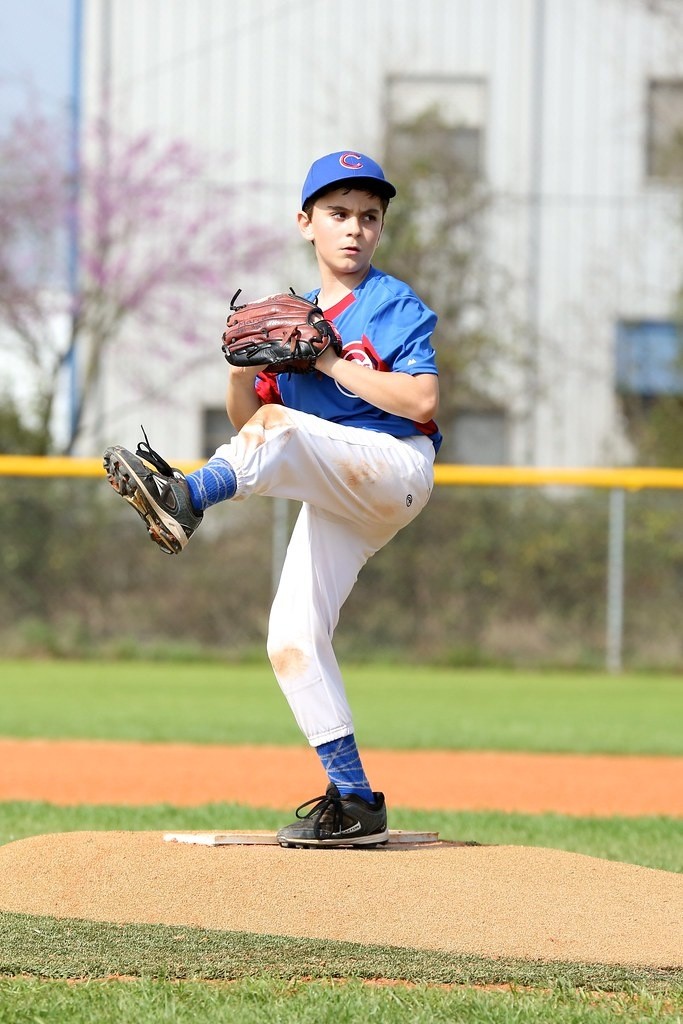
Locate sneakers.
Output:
[103,425,204,555]
[276,782,389,849]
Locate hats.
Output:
[302,150,396,211]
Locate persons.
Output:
[102,151,443,848]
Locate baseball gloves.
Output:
[220,286,343,373]
